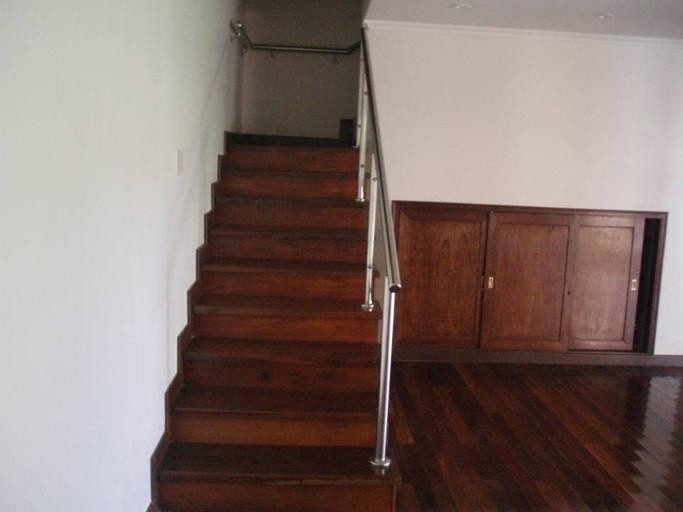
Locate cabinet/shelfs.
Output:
[391,201,487,349]
[479,203,668,355]
